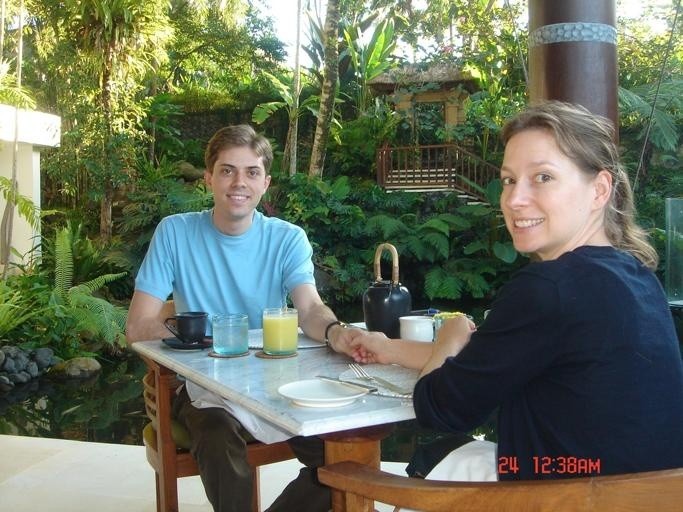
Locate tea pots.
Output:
[361,242,410,340]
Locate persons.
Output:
[124,124,374,512]
[348,99,683,485]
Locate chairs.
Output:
[317,460,682,512]
[127,300,298,512]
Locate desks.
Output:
[131,321,418,512]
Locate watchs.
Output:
[325,320,347,347]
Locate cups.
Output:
[163,312,208,345]
[432,312,472,338]
[211,313,250,356]
[398,314,437,343]
[261,308,300,356]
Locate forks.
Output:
[346,361,414,395]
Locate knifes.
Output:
[313,375,380,395]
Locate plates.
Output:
[160,336,213,352]
[276,378,367,409]
[337,363,420,399]
[246,326,332,350]
